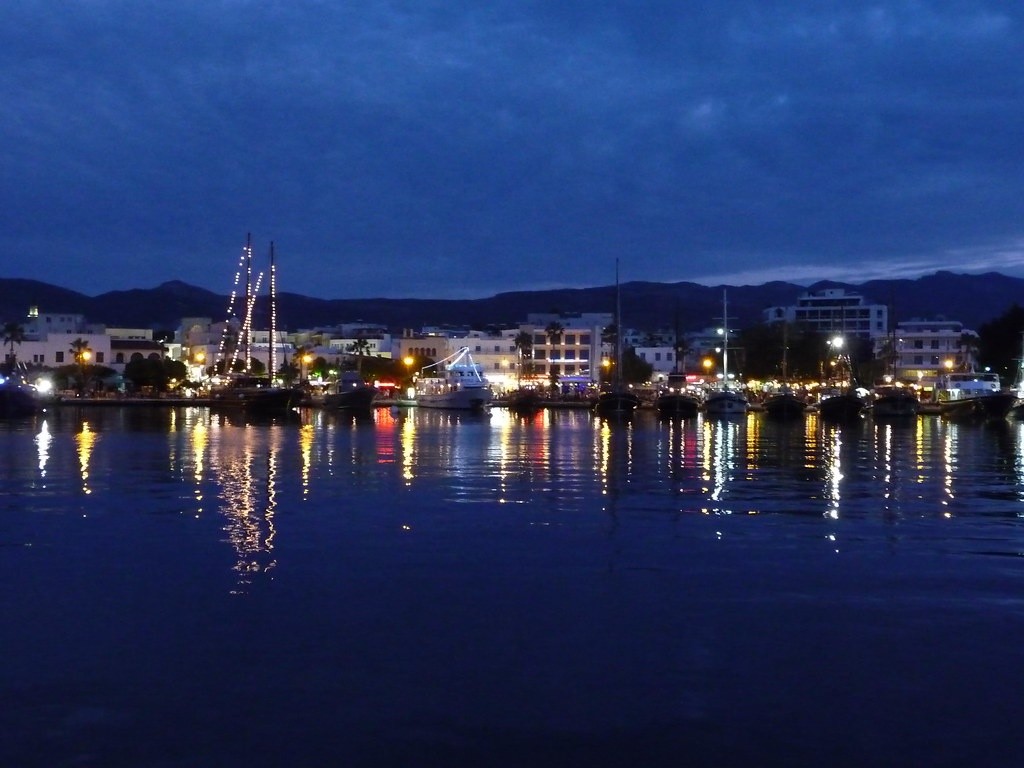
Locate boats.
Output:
[763,393,808,419]
[820,393,866,424]
[592,390,642,413]
[939,393,1019,423]
[653,389,699,419]
[701,385,748,415]
[873,395,919,418]
[323,386,379,414]
[232,388,305,417]
[416,346,495,408]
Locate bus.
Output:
[937,373,1002,399]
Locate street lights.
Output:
[405,356,414,376]
[704,357,716,382]
[602,359,617,381]
[824,336,845,374]
[9,305,39,355]
[77,350,92,390]
[299,354,314,385]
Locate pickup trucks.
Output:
[54,389,79,399]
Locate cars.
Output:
[0,370,38,422]
[140,385,160,396]
